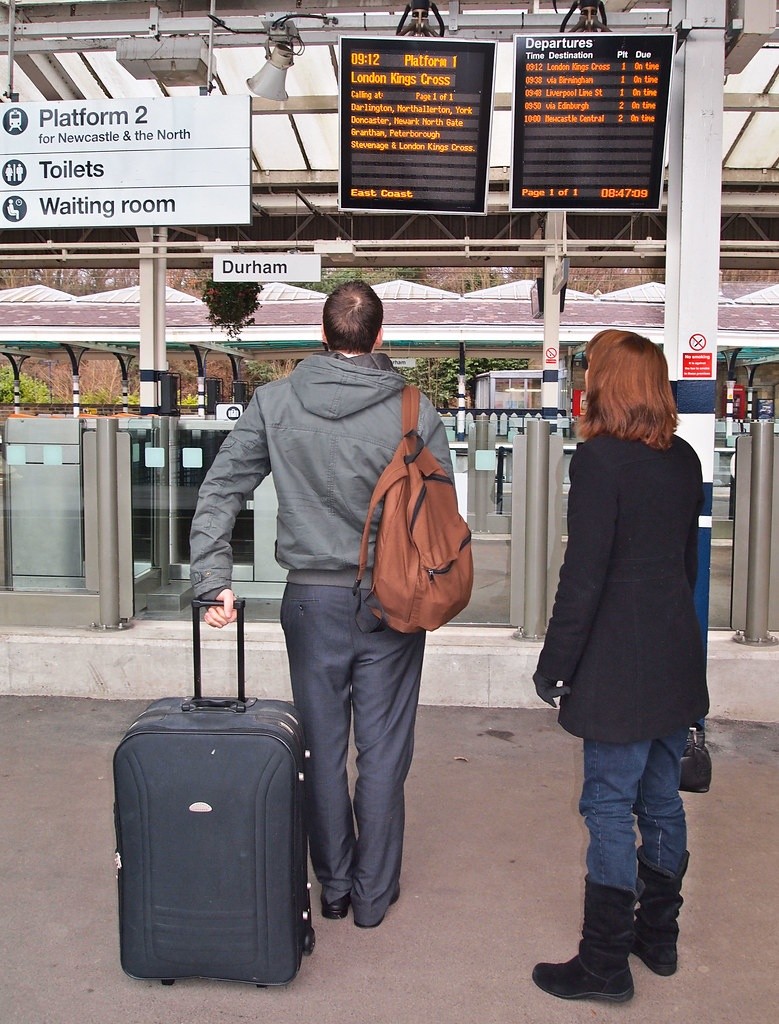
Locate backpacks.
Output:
[354,383,473,636]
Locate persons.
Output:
[190,281,455,927]
[530,331,712,998]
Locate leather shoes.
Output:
[322,893,351,920]
[355,882,401,926]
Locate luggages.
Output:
[112,599,316,985]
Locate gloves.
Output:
[532,673,570,707]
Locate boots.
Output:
[531,873,646,1001]
[631,845,691,976]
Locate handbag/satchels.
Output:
[677,741,715,791]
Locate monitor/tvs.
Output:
[509,32,677,212]
[335,34,499,216]
